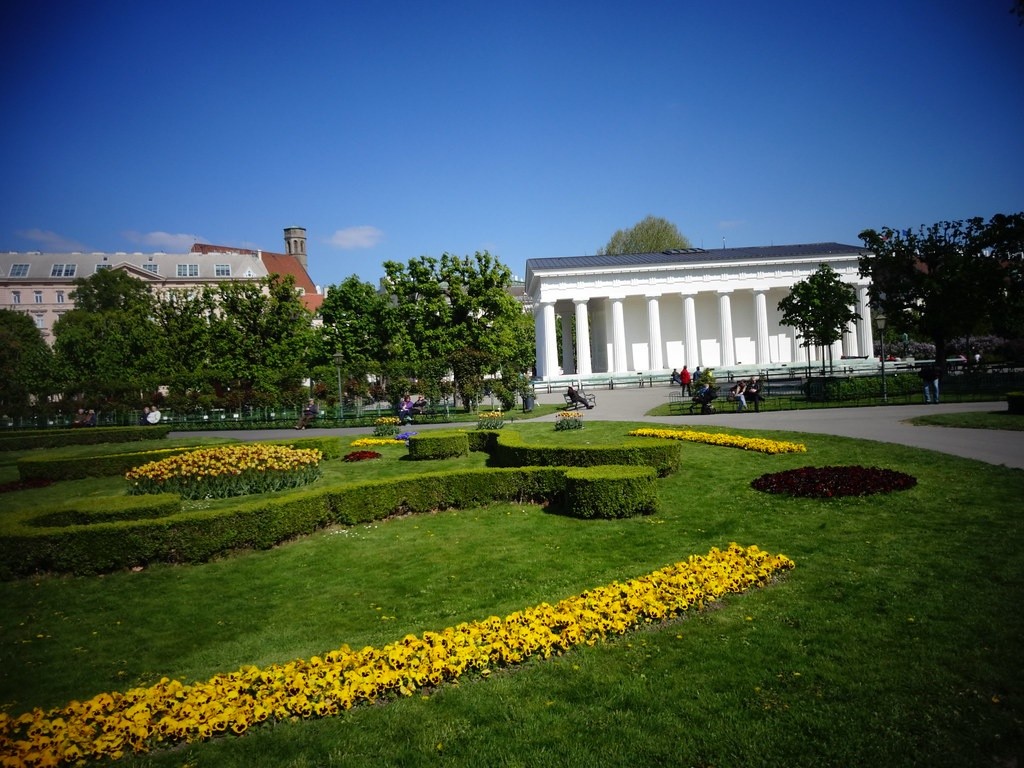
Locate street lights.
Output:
[333,350,345,419]
[874,314,888,403]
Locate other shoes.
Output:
[406,416,412,421]
[689,395,692,397]
[587,405,594,409]
[405,423,411,426]
[755,409,759,412]
[744,406,748,409]
[737,409,741,413]
[400,417,404,420]
[759,397,765,401]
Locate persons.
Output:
[342,392,358,418]
[294,399,320,430]
[693,367,702,381]
[74,409,87,428]
[923,363,945,404]
[567,386,594,409]
[672,369,681,384]
[680,366,692,396]
[731,377,765,414]
[405,396,427,427]
[84,410,97,428]
[694,384,709,398]
[399,395,413,426]
[140,404,162,426]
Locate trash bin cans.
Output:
[524,393,534,409]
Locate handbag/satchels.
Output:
[727,394,735,401]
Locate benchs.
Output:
[563,389,595,411]
[520,358,1024,394]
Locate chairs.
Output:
[0,377,1024,432]
